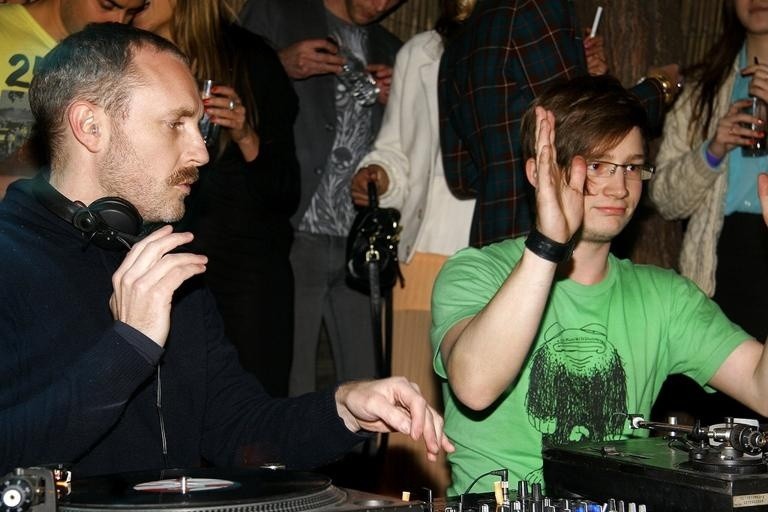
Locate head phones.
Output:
[30,168,144,254]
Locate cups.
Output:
[738,97,767,158]
[195,79,228,147]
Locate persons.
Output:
[1,21,456,496]
[426,74,768,498]
[343,2,476,496]
[234,0,411,402]
[644,0,768,428]
[131,0,306,400]
[433,2,688,251]
[2,0,151,204]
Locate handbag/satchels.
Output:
[344,182,402,296]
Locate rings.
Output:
[727,125,735,134]
[226,100,237,111]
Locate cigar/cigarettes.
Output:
[589,5,604,40]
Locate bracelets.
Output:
[523,226,577,266]
[649,74,674,109]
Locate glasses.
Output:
[586,161,655,180]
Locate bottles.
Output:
[327,38,380,106]
